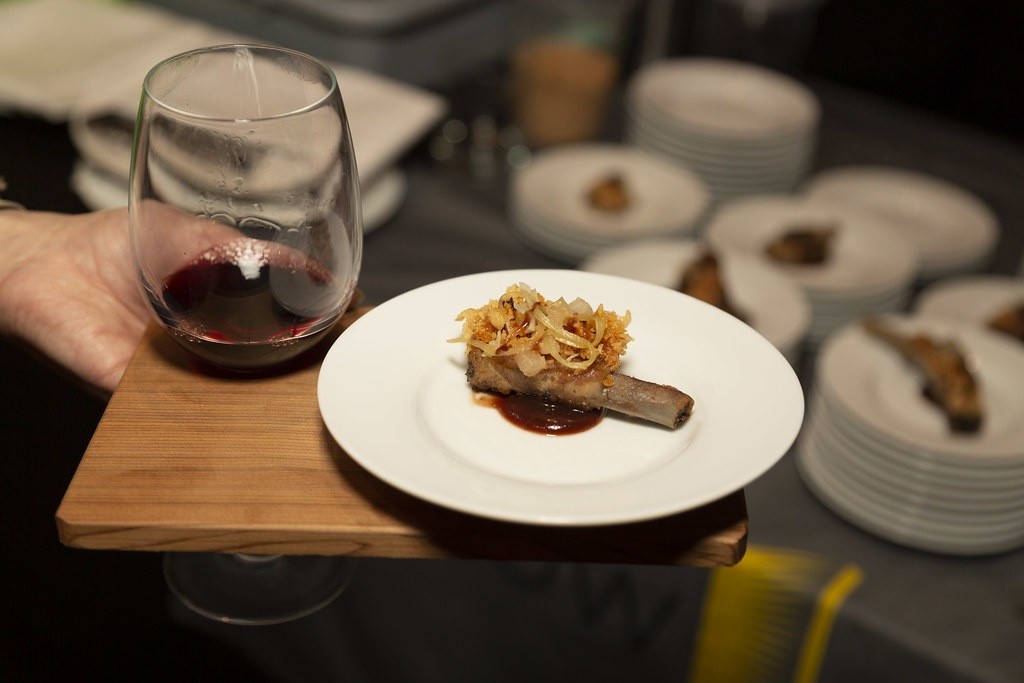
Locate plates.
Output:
[70,59,1024,555]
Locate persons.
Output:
[1,177,363,394]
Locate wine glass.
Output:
[128,46,362,624]
